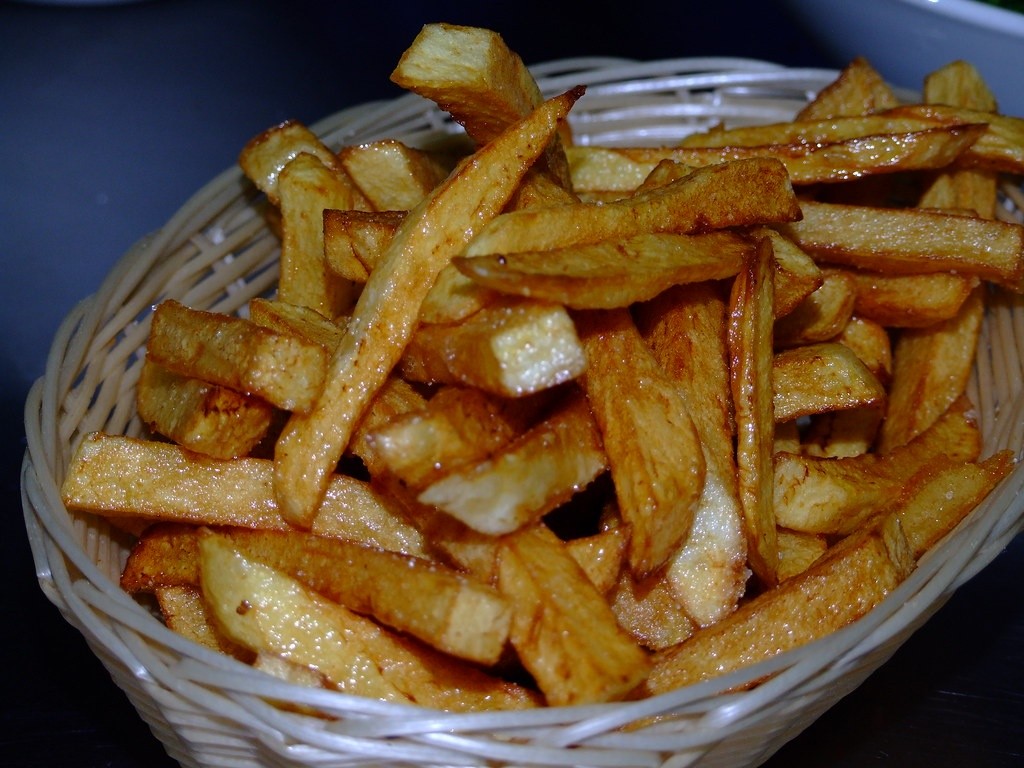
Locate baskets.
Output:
[16,55,1024,768]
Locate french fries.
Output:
[62,23,1024,715]
